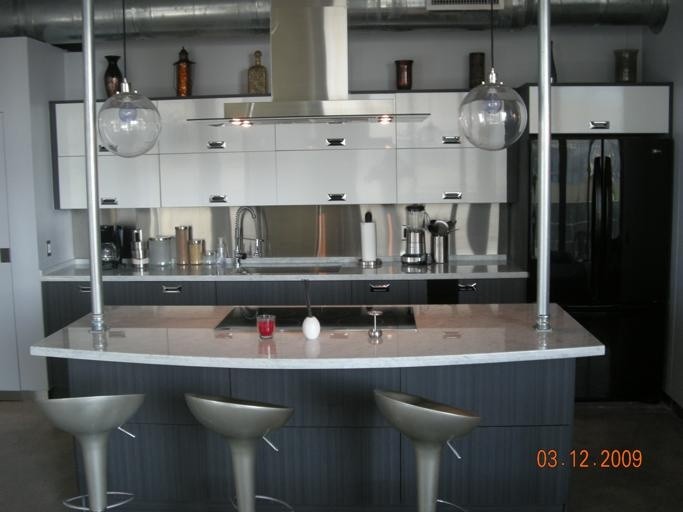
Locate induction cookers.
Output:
[214,306,417,332]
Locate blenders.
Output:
[403,205,430,263]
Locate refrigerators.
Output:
[527,136,674,405]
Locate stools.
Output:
[183,392,297,510]
[43,391,147,509]
[372,390,480,510]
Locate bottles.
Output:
[103,56,124,101]
[174,44,194,98]
[550,41,556,85]
[247,50,268,95]
[395,60,414,92]
[148,226,226,267]
[615,49,639,84]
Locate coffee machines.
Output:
[100,225,121,270]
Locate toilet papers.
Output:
[359,220,377,263]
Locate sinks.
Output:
[244,262,342,275]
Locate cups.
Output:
[256,315,277,340]
[469,52,485,89]
[432,234,449,264]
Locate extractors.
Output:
[184,0,432,124]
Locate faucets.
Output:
[233,206,256,266]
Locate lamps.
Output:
[456,2,530,151]
[97,2,159,157]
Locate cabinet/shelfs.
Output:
[510,80,670,136]
[44,283,215,397]
[353,280,524,306]
[49,88,509,208]
